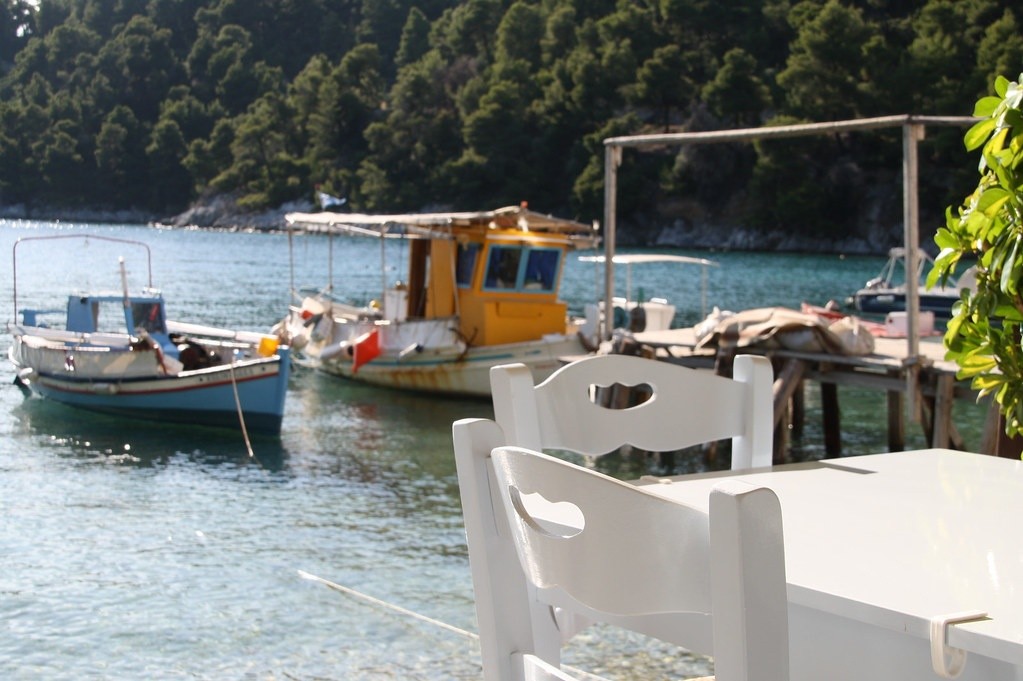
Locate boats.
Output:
[575,254,726,368]
[847,246,1001,330]
[267,199,602,395]
[7,234,292,437]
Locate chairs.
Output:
[490,353,776,470]
[452,416,787,681]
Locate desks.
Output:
[533,445,1023,681]
